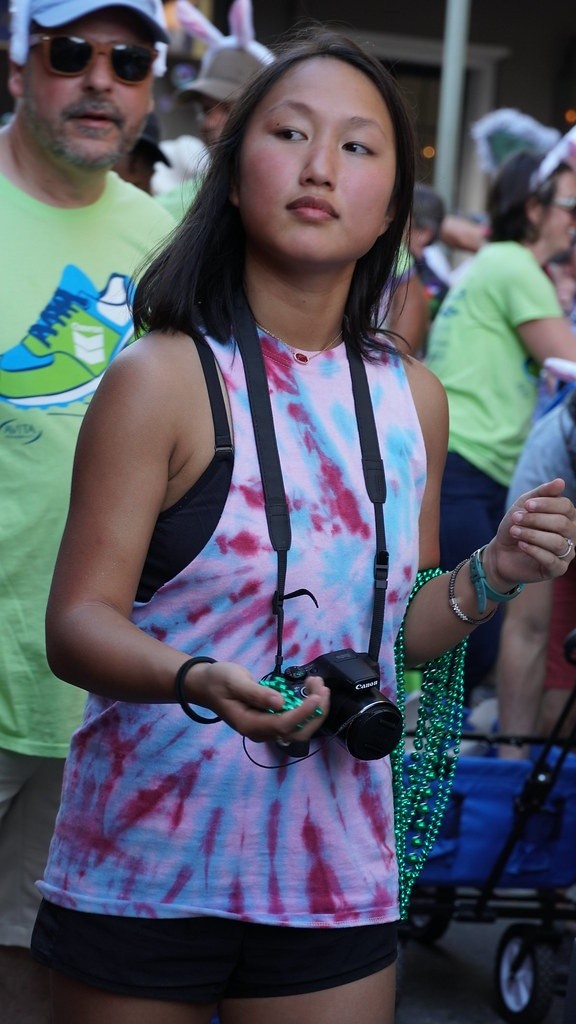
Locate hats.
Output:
[175,0,277,100]
[147,135,211,193]
[134,112,173,171]
[27,0,173,44]
[468,107,561,187]
[410,183,445,248]
[470,128,576,218]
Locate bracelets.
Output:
[448,559,497,624]
[470,544,524,615]
[176,656,223,724]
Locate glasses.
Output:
[29,33,160,85]
[550,197,576,215]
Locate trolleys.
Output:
[390,629,576,1024]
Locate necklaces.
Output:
[393,569,472,921]
[256,322,343,364]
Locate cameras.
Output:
[282,649,402,762]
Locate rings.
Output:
[557,538,574,559]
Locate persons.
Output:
[28,34,576,1024]
[368,108,576,762]
[0,0,280,1024]
